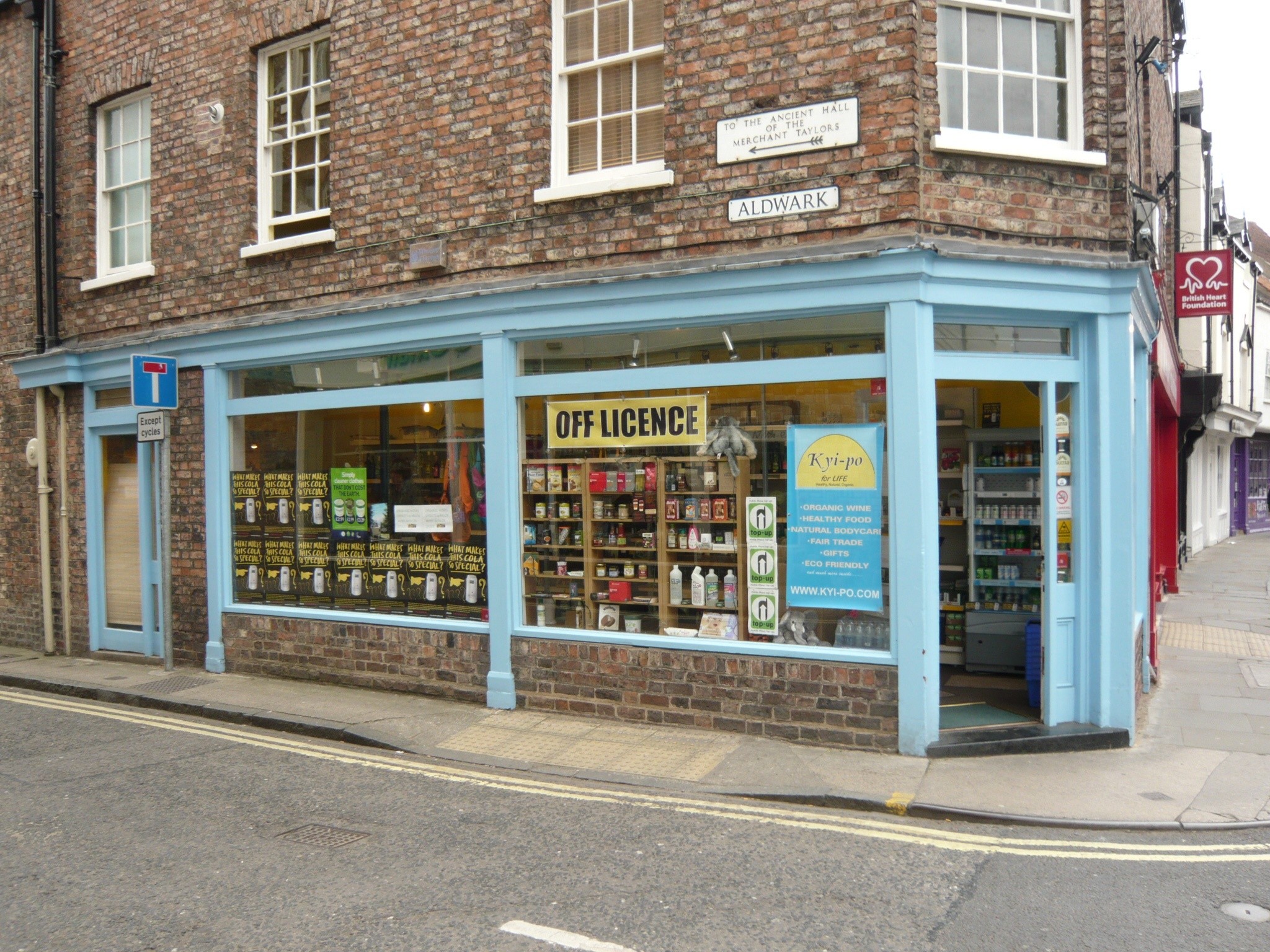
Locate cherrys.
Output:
[942,453,959,470]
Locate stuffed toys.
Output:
[696,415,758,477]
[772,608,832,646]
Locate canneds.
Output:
[426,573,437,600]
[728,497,736,519]
[279,499,289,524]
[387,571,397,598]
[535,502,582,519]
[313,499,324,524]
[351,569,362,596]
[248,565,258,590]
[976,476,1041,520]
[677,462,718,492]
[618,504,629,520]
[595,561,648,579]
[465,575,477,603]
[592,533,605,547]
[603,504,615,519]
[280,566,290,592]
[246,498,255,522]
[314,568,324,593]
[542,527,583,597]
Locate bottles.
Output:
[667,523,678,549]
[537,599,546,626]
[623,614,642,633]
[1032,527,1041,550]
[691,565,705,606]
[705,568,719,606]
[833,616,890,650]
[990,441,1041,467]
[678,529,688,549]
[724,569,738,608]
[975,528,1026,549]
[608,524,617,547]
[424,451,438,478]
[670,565,683,605]
[616,523,627,547]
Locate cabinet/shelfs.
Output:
[965,426,1043,676]
[522,416,965,666]
[351,434,460,487]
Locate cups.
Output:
[557,562,567,576]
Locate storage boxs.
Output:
[606,470,617,491]
[1024,616,1042,705]
[700,498,712,520]
[625,472,635,492]
[568,464,581,491]
[526,464,545,491]
[713,498,729,520]
[547,465,564,492]
[597,603,620,631]
[609,581,632,601]
[683,497,699,520]
[617,472,625,491]
[665,499,680,520]
[590,470,606,492]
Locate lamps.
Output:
[619,356,627,369]
[824,342,835,356]
[531,359,540,376]
[585,358,593,372]
[871,338,883,352]
[719,327,741,362]
[628,335,644,367]
[701,348,710,364]
[768,344,778,360]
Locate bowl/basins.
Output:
[664,627,698,637]
